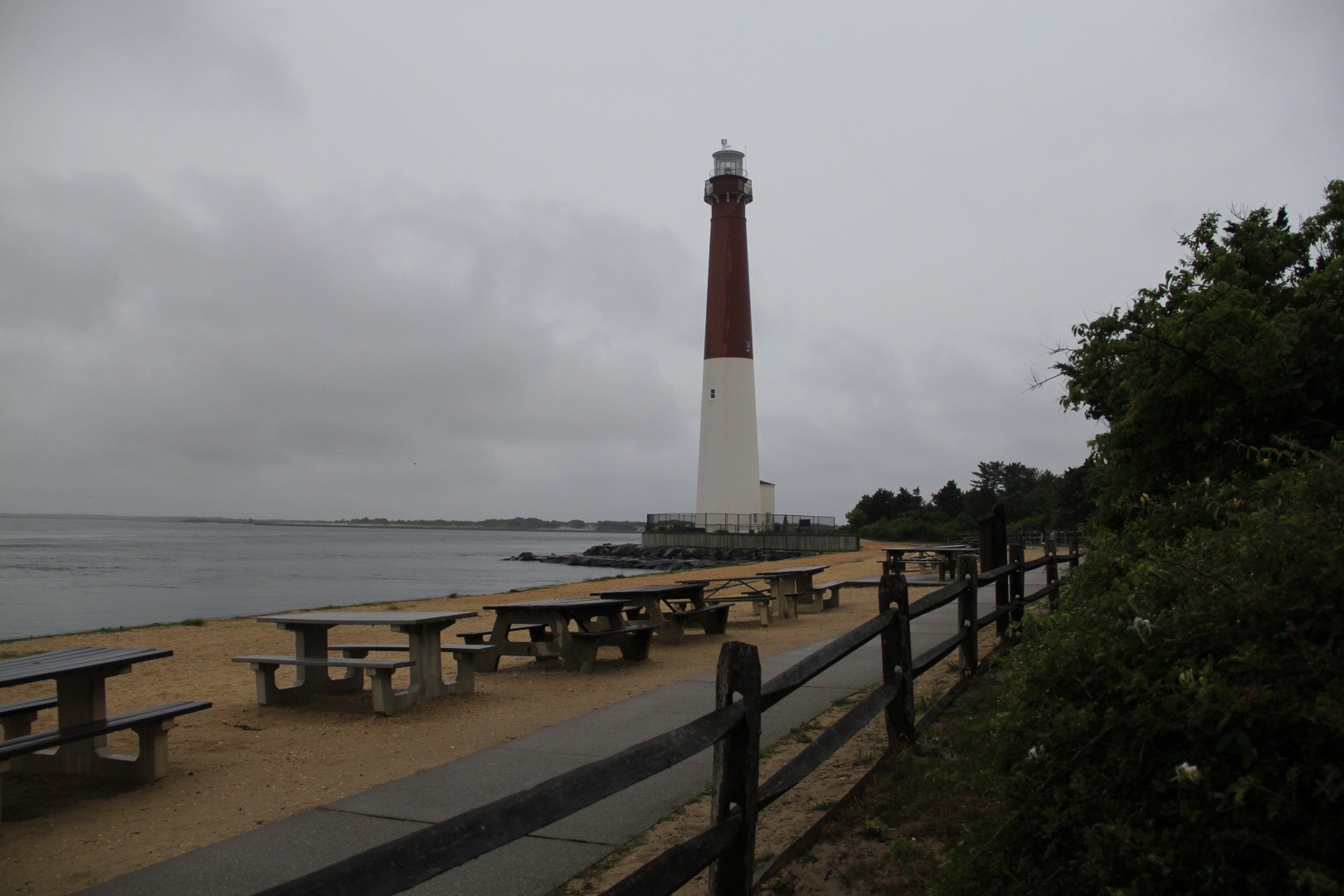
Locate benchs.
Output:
[455,624,548,672]
[0,699,213,782]
[230,655,416,715]
[808,580,846,612]
[622,607,642,620]
[741,587,772,612]
[0,696,58,739]
[784,592,804,617]
[668,596,776,627]
[876,559,956,581]
[912,554,940,560]
[568,624,659,671]
[327,642,494,700]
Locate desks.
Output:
[674,574,787,612]
[756,566,830,608]
[590,583,710,645]
[0,645,173,782]
[250,610,477,715]
[482,598,633,673]
[881,546,979,581]
